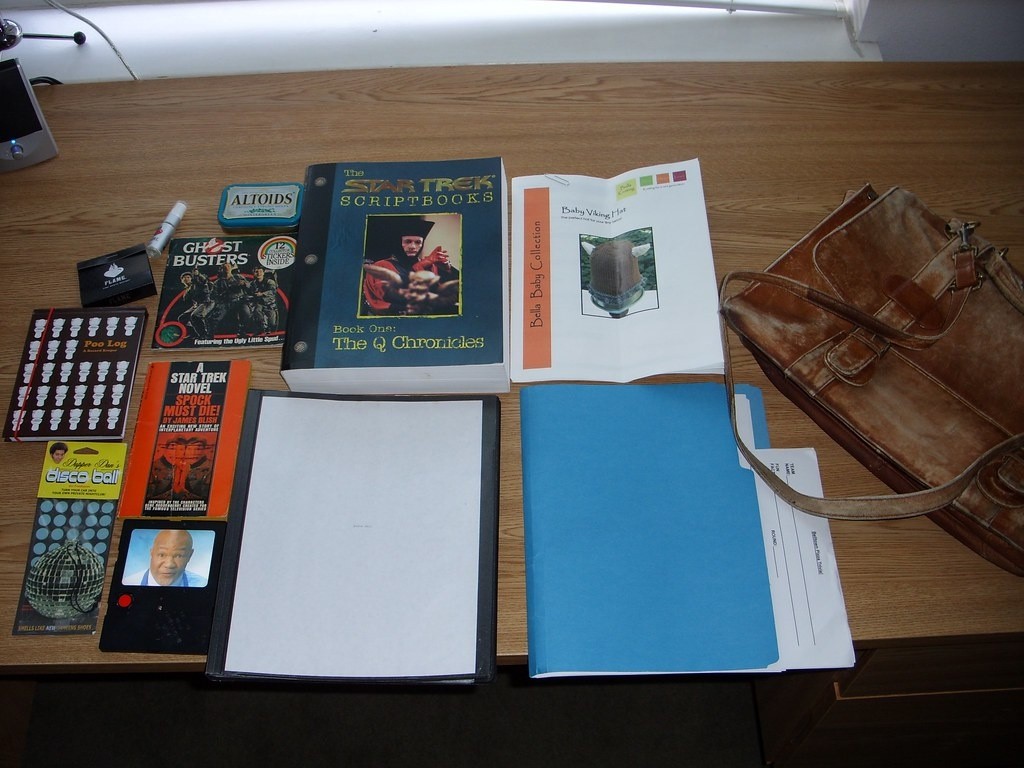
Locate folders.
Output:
[522,379,793,686]
[203,388,501,690]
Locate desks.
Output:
[0,61,1024,768]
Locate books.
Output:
[148,233,298,351]
[117,359,252,524]
[2,307,149,442]
[11,499,118,635]
[205,389,501,691]
[278,157,512,396]
[509,158,724,386]
[37,439,128,500]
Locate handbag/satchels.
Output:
[723,184,1023,593]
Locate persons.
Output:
[179,263,278,342]
[364,220,459,315]
[121,530,209,586]
